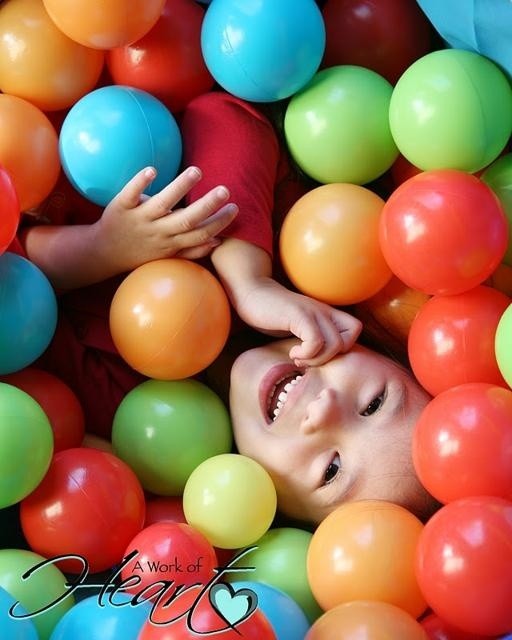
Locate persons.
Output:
[1,0,512,640]
